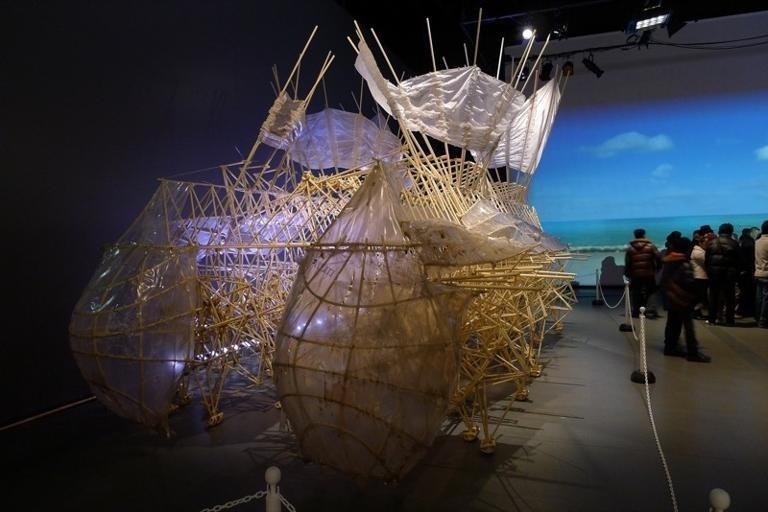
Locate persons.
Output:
[623,228,661,321]
[656,238,713,362]
[664,220,768,329]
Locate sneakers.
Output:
[694,312,768,328]
[663,344,712,362]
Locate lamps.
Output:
[510,49,606,81]
